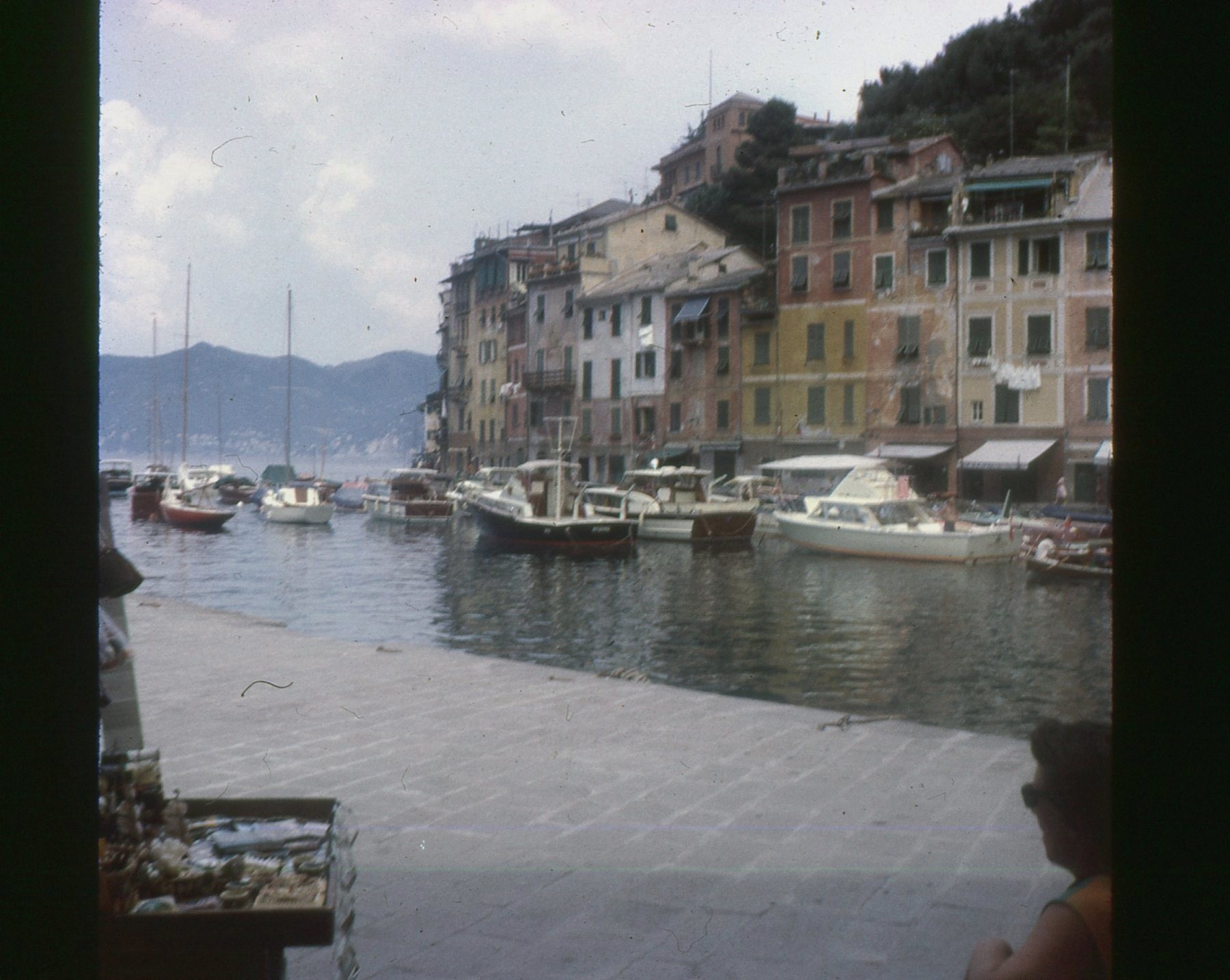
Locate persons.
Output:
[964,720,1111,979]
[1054,473,1067,505]
[941,498,956,533]
[1023,516,1112,571]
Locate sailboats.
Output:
[126,263,344,525]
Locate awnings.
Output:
[867,442,954,461]
[759,454,889,472]
[1094,439,1113,468]
[957,438,1064,469]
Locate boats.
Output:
[258,487,336,525]
[362,467,454,519]
[579,458,762,542]
[98,458,134,496]
[460,415,640,547]
[1021,459,1114,582]
[445,465,517,515]
[709,472,805,529]
[328,477,387,509]
[767,463,1024,563]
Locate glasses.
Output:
[1021,783,1050,808]
